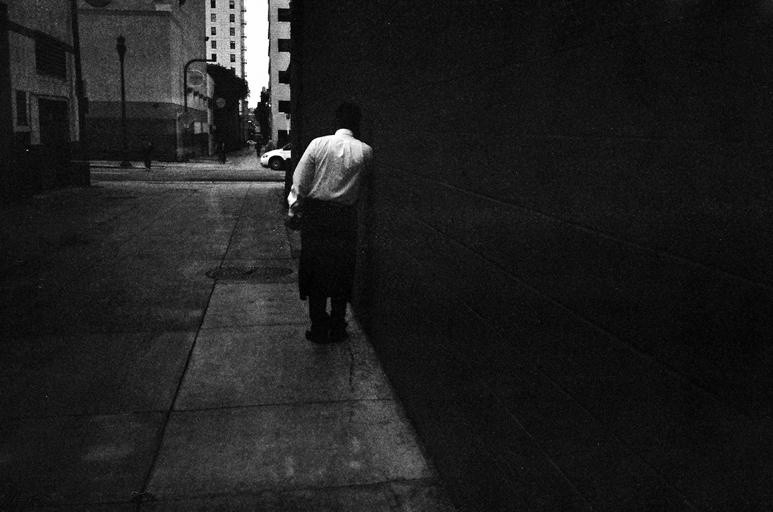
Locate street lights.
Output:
[115,33,135,168]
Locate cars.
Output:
[259,143,292,170]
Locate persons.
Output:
[286,105,375,345]
